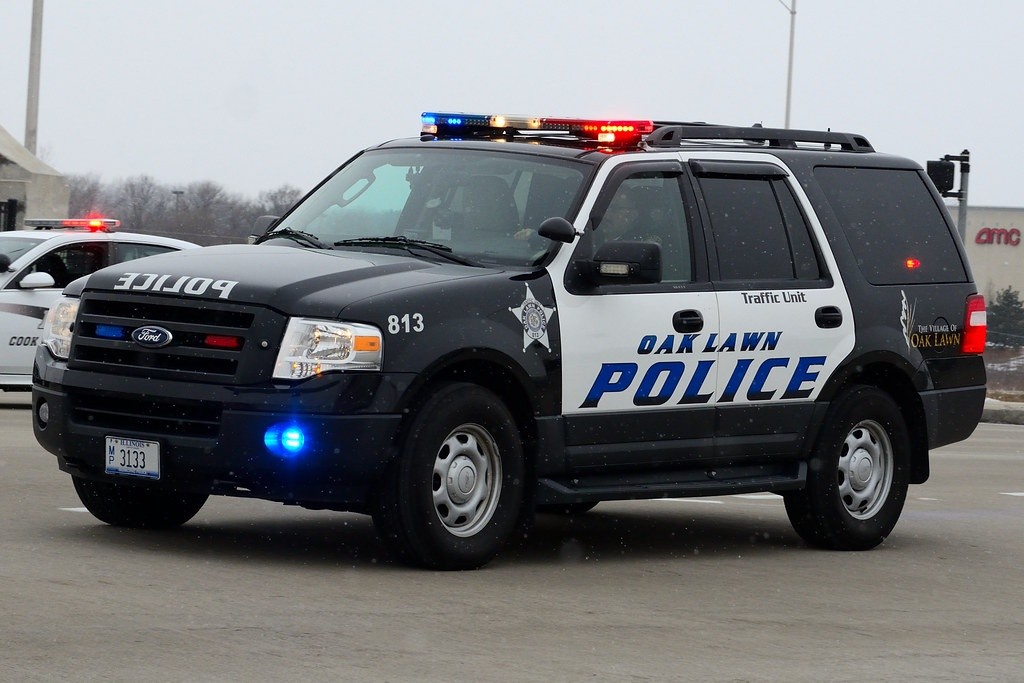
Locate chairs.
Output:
[633,185,685,280]
[452,175,521,249]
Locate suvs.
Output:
[28,111,988,573]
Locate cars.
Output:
[0,217,206,390]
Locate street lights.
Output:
[171,190,184,223]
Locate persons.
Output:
[513,187,669,269]
